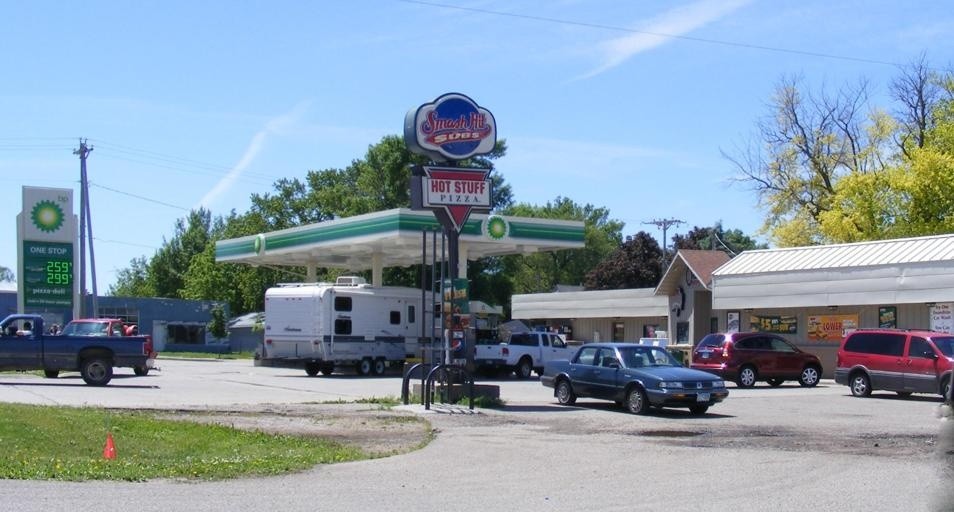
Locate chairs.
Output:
[603,357,615,368]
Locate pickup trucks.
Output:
[0,315,156,387]
[473,332,579,380]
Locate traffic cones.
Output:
[103,432,119,461]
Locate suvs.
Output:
[692,332,823,390]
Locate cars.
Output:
[540,343,729,417]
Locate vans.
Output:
[834,328,953,404]
